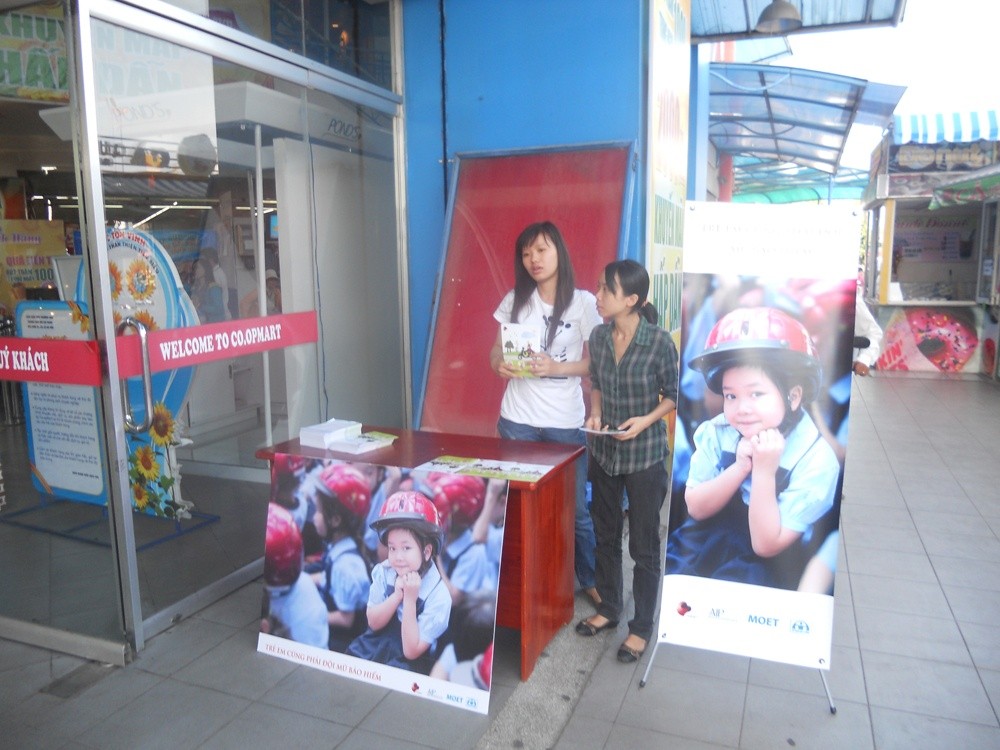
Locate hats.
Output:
[263,269,277,280]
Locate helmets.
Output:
[472,641,493,690]
[310,464,371,517]
[369,471,484,554]
[272,454,303,484]
[686,307,814,392]
[264,501,302,597]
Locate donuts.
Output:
[882,306,980,376]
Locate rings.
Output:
[862,371,865,376]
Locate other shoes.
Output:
[575,615,619,636]
[583,588,602,610]
[617,627,653,663]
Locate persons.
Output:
[179,249,231,328]
[576,259,678,664]
[855,295,886,377]
[489,220,604,614]
[664,260,852,597]
[261,451,508,692]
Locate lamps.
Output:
[754,0,802,37]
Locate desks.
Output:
[254,425,588,681]
